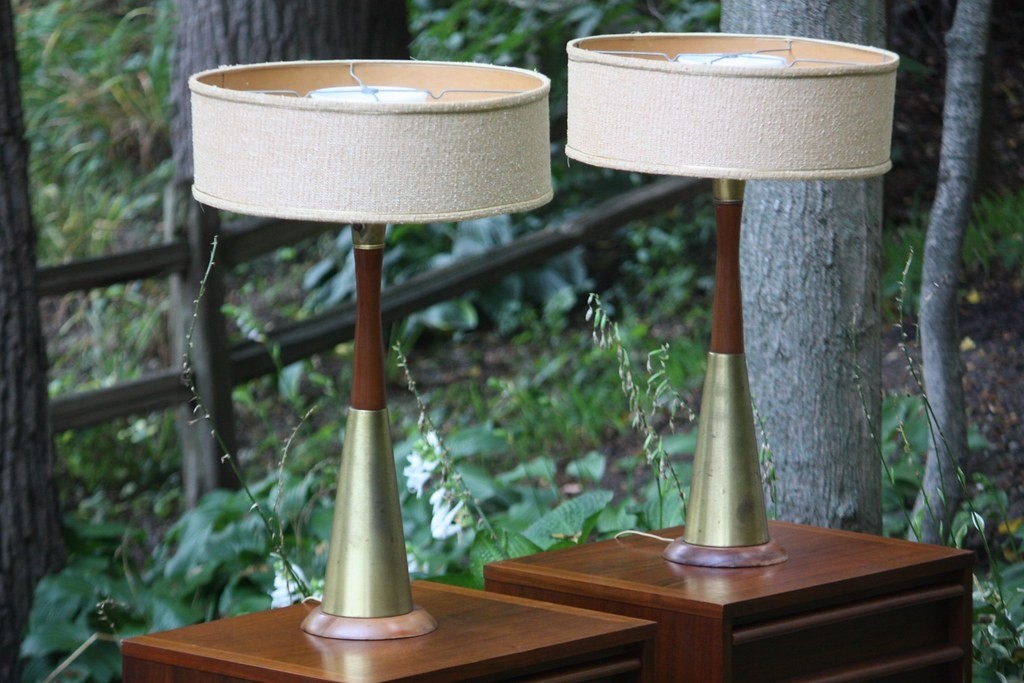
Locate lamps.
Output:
[565,33,901,570]
[186,57,553,641]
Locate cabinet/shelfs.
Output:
[482,519,974,683]
[121,578,658,683]
